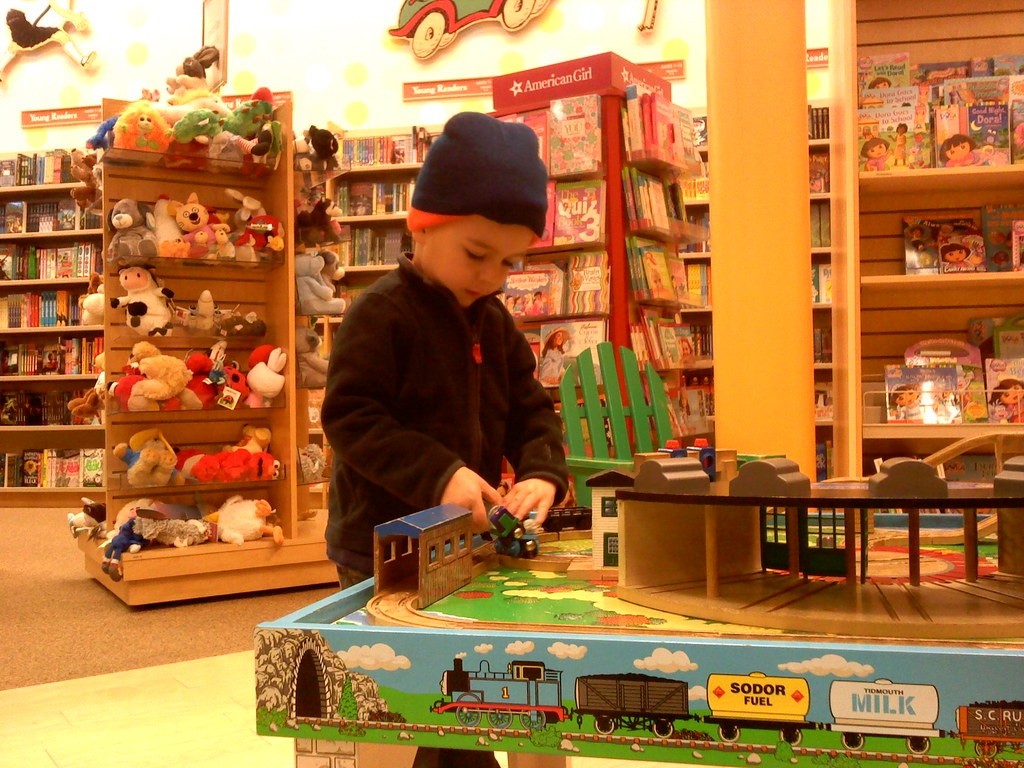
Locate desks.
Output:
[252,512,1024,768]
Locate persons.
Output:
[321,112,570,591]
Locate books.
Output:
[0,146,106,491]
[900,198,1024,277]
[885,358,1024,424]
[857,50,1024,170]
[496,85,832,443]
[333,125,443,317]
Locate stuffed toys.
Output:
[65,263,288,580]
[84,46,345,173]
[105,188,343,264]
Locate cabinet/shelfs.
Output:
[0,0,1024,607]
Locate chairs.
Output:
[558,341,674,508]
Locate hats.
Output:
[407,112,548,249]
